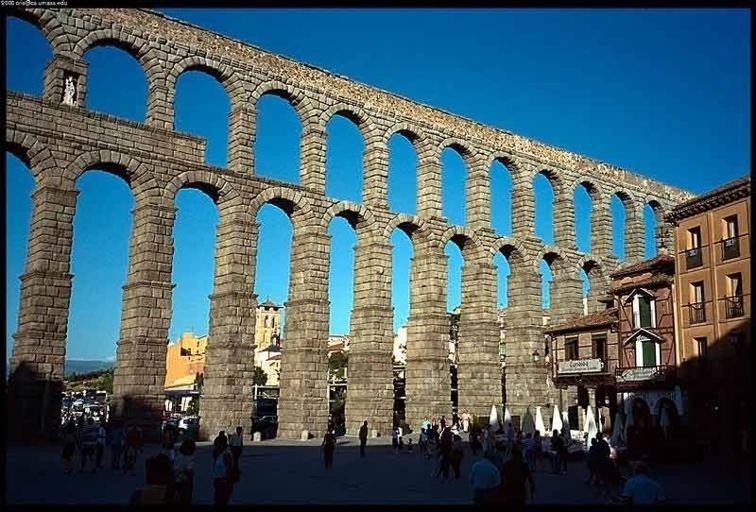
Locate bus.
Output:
[71,398,106,428]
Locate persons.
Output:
[185,347,193,355]
[357,419,369,458]
[388,409,756,512]
[47,404,243,508]
[319,424,336,471]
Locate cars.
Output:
[162,401,278,438]
